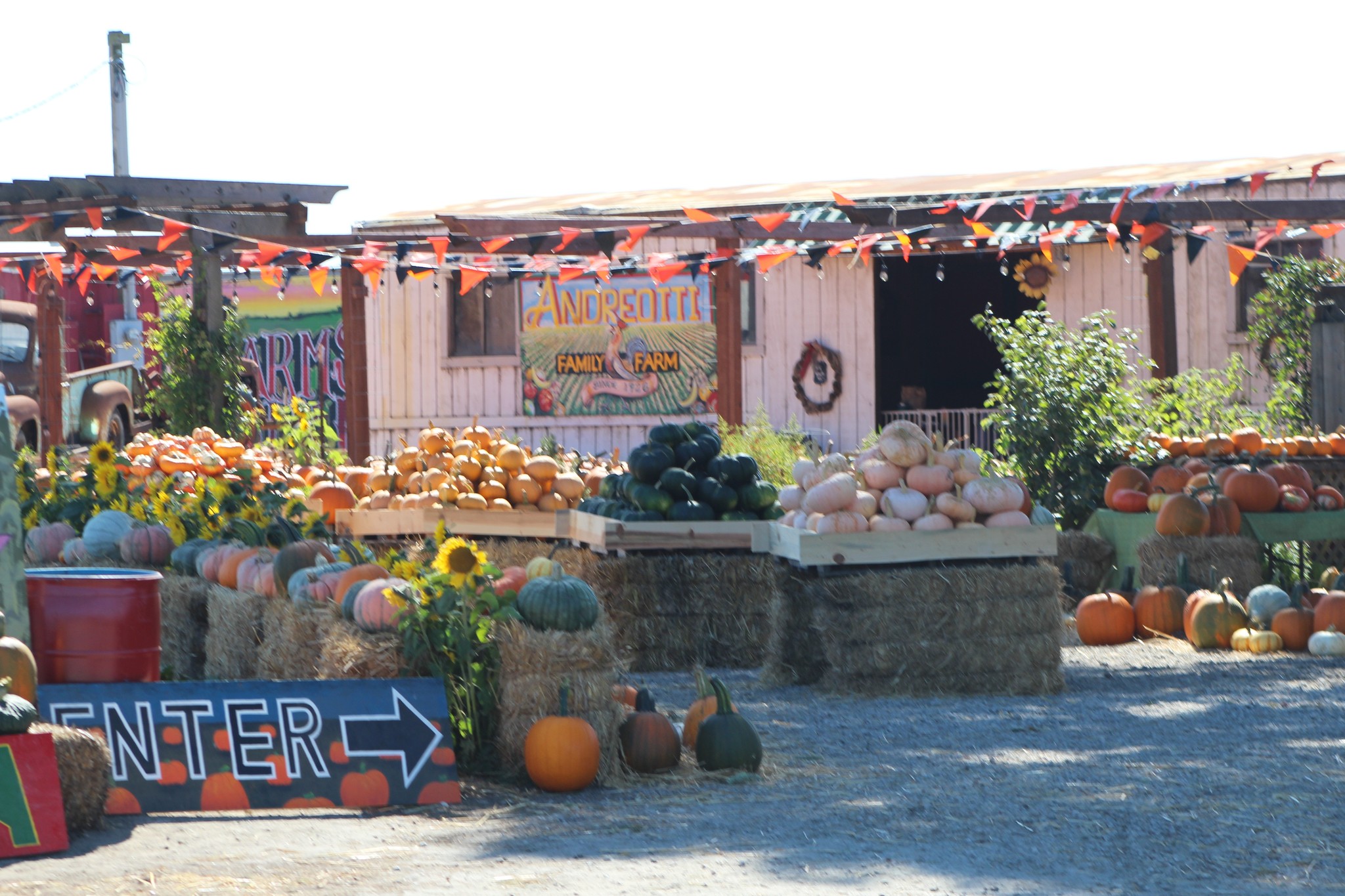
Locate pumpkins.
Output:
[1,418,1344,840]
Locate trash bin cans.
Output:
[23,566,164,684]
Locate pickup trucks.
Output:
[0,298,157,461]
